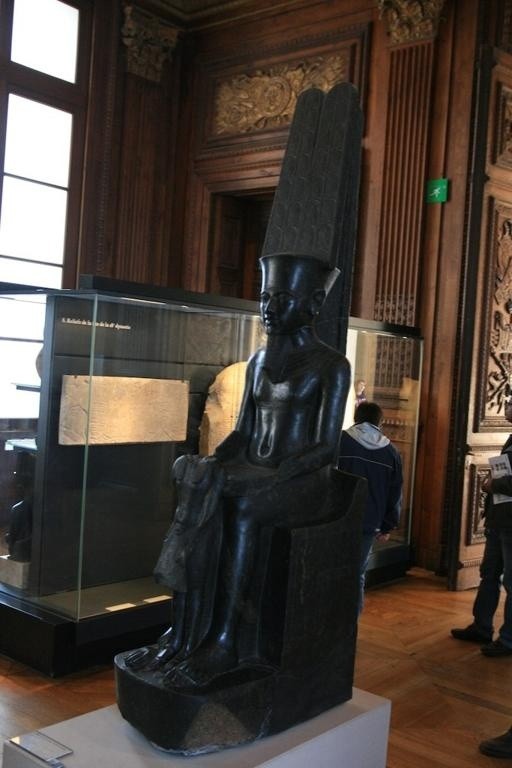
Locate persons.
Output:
[451,395,512,657]
[337,398,404,619]
[153,255,351,673]
[478,723,512,760]
[354,379,367,407]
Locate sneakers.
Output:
[479,640,512,657]
[479,728,511,759]
[450,623,494,644]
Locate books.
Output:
[488,454,512,505]
